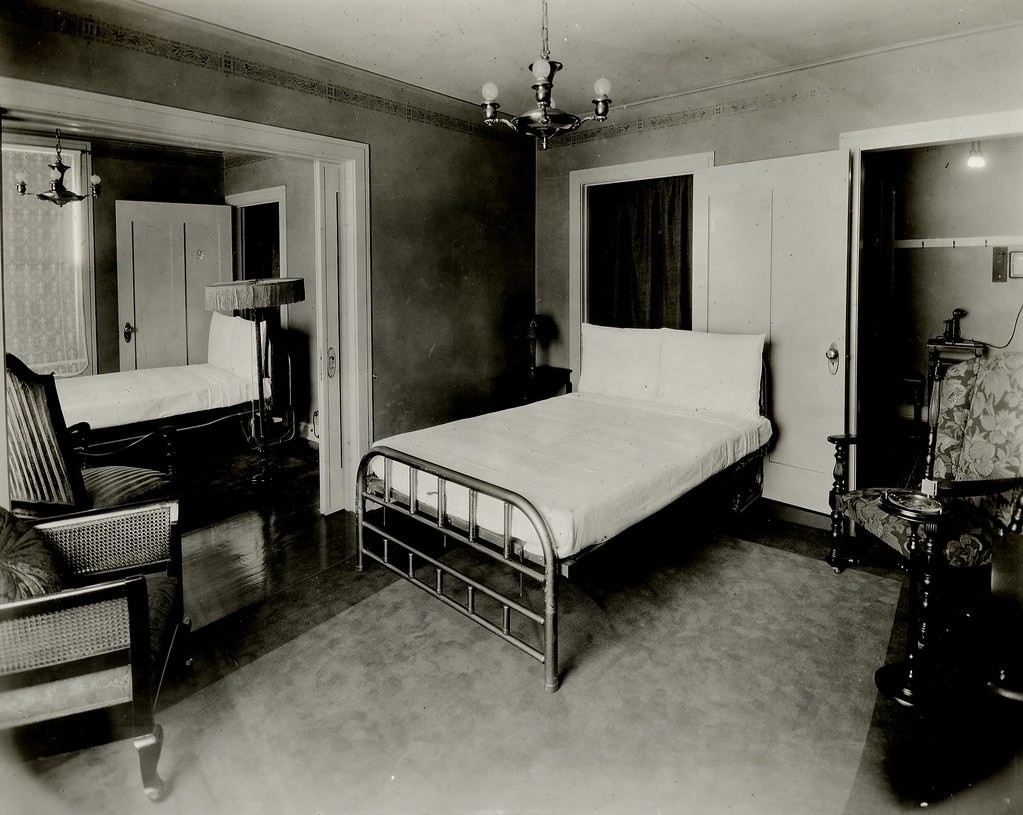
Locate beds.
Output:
[54,311,273,454]
[355,321,779,695]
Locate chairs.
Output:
[0,495,200,803]
[827,351,1023,652]
[5,352,177,518]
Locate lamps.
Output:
[200,275,307,489]
[17,126,101,207]
[481,0,613,150]
